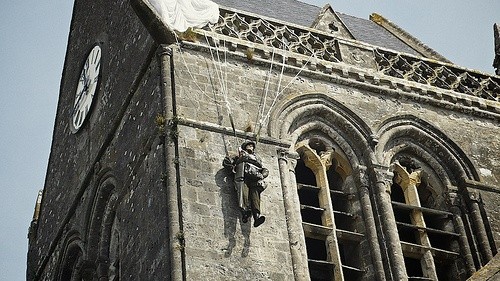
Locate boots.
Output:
[253,213,266,227]
[241,209,252,223]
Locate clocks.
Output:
[68,40,104,134]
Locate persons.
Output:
[224,141,268,226]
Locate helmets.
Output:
[242,142,255,150]
